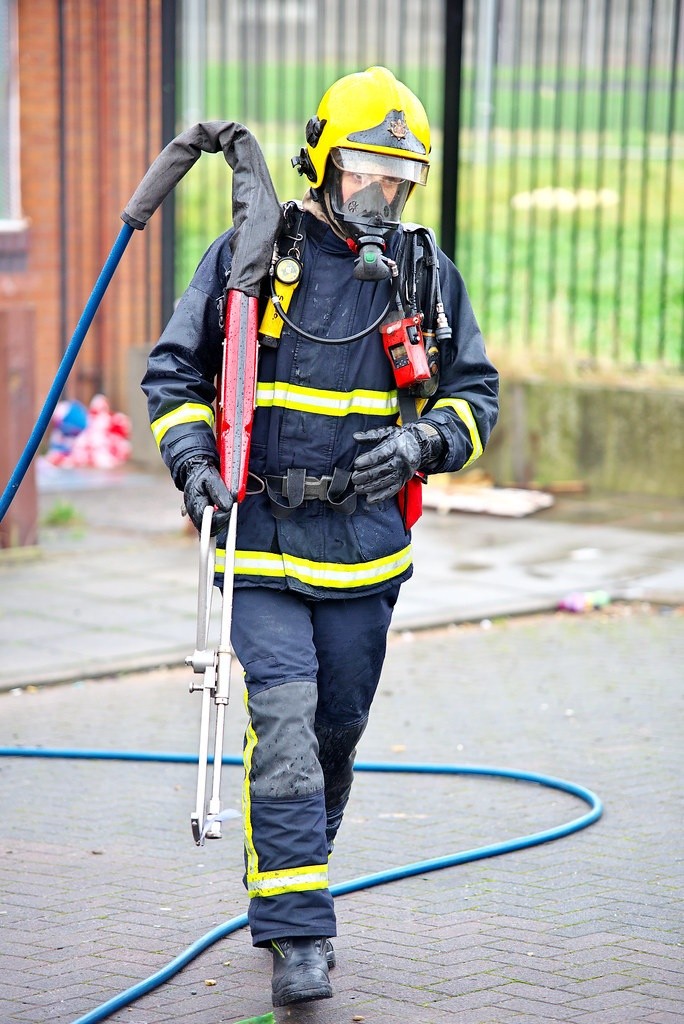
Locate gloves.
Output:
[348,422,446,504]
[175,455,235,537]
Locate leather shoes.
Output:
[267,935,337,1008]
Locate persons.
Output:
[142,66,500,1007]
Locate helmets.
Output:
[291,66,434,190]
